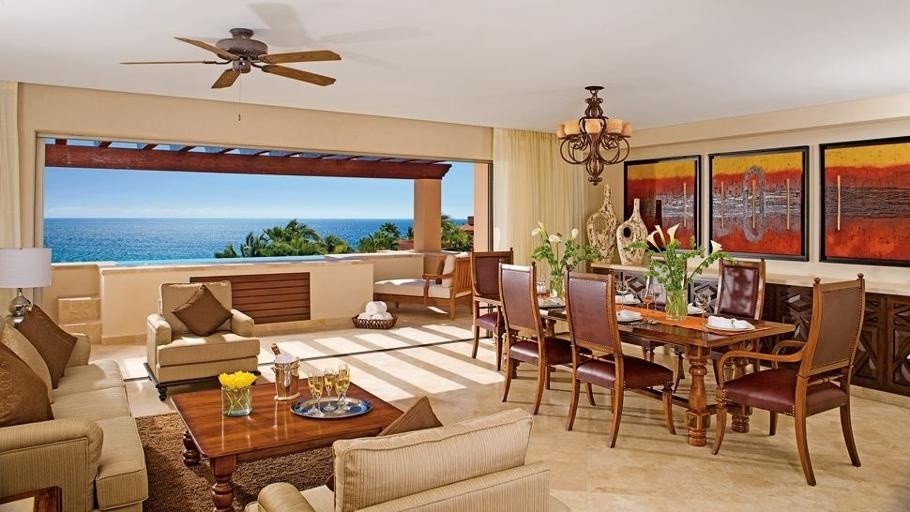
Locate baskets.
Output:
[353,314,396,329]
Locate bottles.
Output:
[271,343,290,363]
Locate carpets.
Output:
[133,411,332,512]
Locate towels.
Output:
[356,300,393,328]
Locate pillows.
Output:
[0,343,54,428]
[323,396,445,493]
[435,255,445,286]
[0,324,56,404]
[169,284,234,337]
[14,303,78,390]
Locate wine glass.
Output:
[695,293,711,325]
[650,284,663,313]
[616,281,630,311]
[307,364,352,416]
[641,289,654,318]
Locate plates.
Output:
[705,322,756,332]
[617,310,642,323]
[615,298,640,306]
[687,311,707,315]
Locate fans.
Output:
[120,27,341,88]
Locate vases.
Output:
[221,385,253,417]
[586,183,620,264]
[614,198,648,266]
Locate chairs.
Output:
[671,256,766,394]
[711,272,865,486]
[563,265,677,448]
[244,393,571,511]
[470,246,557,372]
[144,280,261,400]
[645,255,686,307]
[497,257,596,416]
[373,250,472,320]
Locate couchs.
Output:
[0,307,151,510]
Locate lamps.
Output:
[0,246,53,325]
[557,86,635,184]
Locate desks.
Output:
[474,294,796,447]
[0,485,64,511]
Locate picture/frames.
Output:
[622,153,702,253]
[706,145,810,262]
[817,137,910,267]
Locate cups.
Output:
[276,356,300,399]
[359,300,392,320]
[536,282,547,301]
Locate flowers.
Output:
[215,370,258,415]
[531,220,606,292]
[644,222,735,314]
